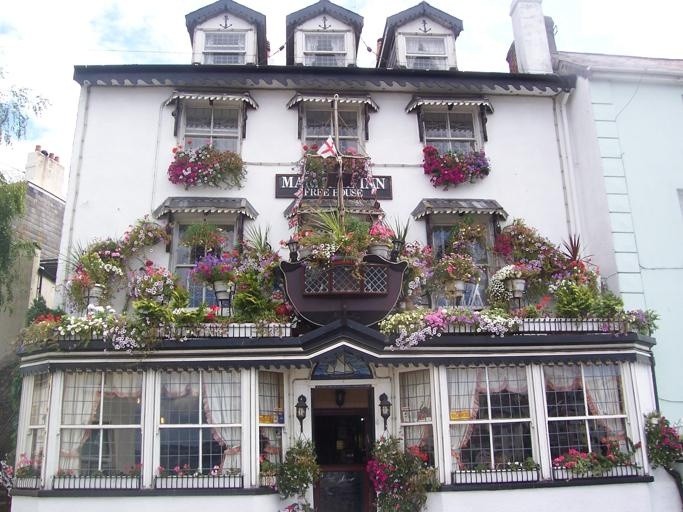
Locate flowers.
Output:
[4,452,282,481]
[366,435,442,512]
[553,409,683,478]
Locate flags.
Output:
[316,135,338,159]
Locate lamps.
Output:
[294,394,308,432]
[379,393,392,430]
